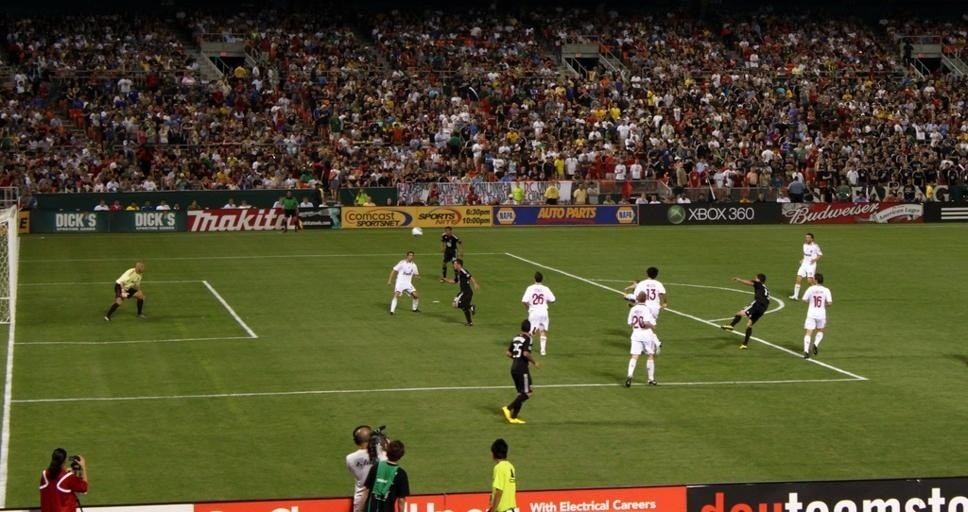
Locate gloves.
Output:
[121,289,130,299]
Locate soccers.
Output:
[412,228,423,236]
[668,205,686,224]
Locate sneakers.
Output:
[501,405,512,421]
[104,315,112,322]
[804,351,811,358]
[810,343,818,355]
[647,379,657,386]
[624,378,631,388]
[464,321,473,326]
[540,350,546,356]
[412,309,423,313]
[439,277,446,283]
[739,343,747,350]
[390,311,395,316]
[789,295,799,301]
[509,417,527,424]
[137,312,147,319]
[720,324,736,330]
[470,304,476,315]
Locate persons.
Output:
[626,265,669,350]
[522,271,556,356]
[103,260,146,322]
[345,425,383,511]
[39,447,89,511]
[801,274,833,358]
[485,439,522,512]
[624,279,640,309]
[439,227,463,284]
[787,233,825,301]
[626,290,659,387]
[502,318,538,425]
[386,250,421,315]
[442,259,479,326]
[360,440,409,511]
[275,189,298,232]
[720,272,769,350]
[2,2,968,215]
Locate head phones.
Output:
[353,425,371,445]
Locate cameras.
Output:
[68,456,81,471]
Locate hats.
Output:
[0,0,967,204]
